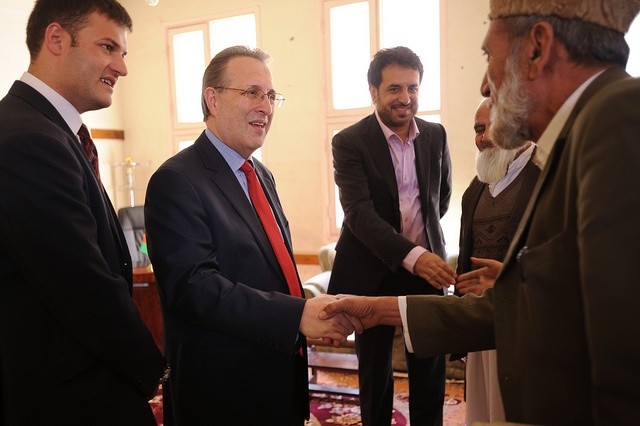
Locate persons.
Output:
[147,46,362,426]
[457,99,540,426]
[321,0,640,424]
[2,0,165,426]
[327,47,459,426]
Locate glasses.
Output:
[211,86,285,106]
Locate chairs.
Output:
[118,206,153,286]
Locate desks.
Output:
[129,267,165,356]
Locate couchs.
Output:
[302,245,459,372]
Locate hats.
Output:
[490,1,639,32]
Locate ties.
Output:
[240,161,302,356]
[78,123,104,193]
